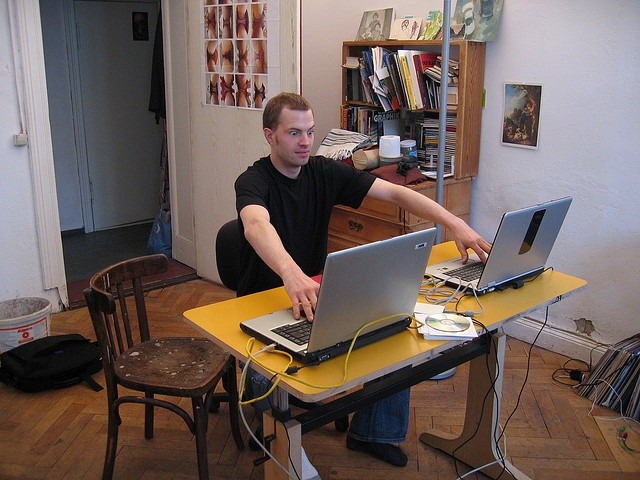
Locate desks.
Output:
[181,240,590,479]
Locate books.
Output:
[573,333,640,423]
[341,46,459,112]
[340,105,457,170]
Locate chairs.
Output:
[212,217,241,297]
[82,251,247,480]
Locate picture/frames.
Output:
[497,82,543,151]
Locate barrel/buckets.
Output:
[0,297,52,353]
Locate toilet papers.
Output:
[379,135,400,158]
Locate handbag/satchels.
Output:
[0,333,103,394]
[146,217,171,258]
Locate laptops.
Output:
[237,226,439,368]
[425,195,574,297]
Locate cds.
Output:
[424,312,471,333]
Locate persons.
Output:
[234,92,493,469]
[369,12,380,32]
[361,27,373,41]
[372,23,381,41]
[204,0,268,108]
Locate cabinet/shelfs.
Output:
[341,39,486,180]
[325,178,472,254]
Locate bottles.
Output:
[400,140,418,159]
